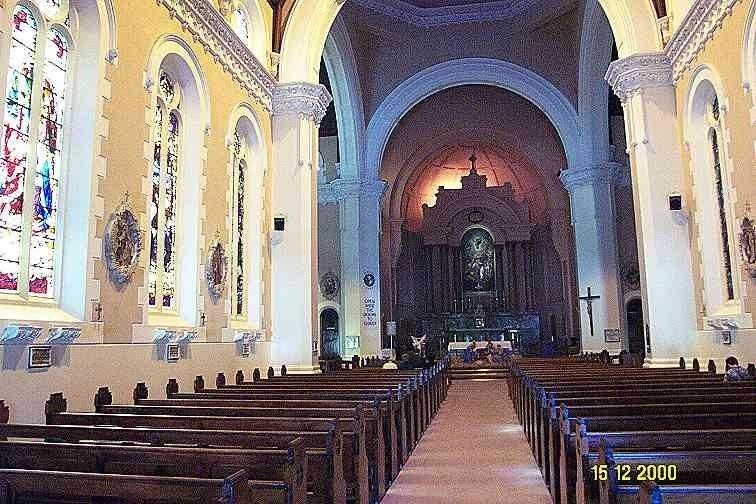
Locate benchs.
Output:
[506,350,756,504]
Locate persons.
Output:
[464,340,476,362]
[486,339,497,361]
[723,356,752,384]
